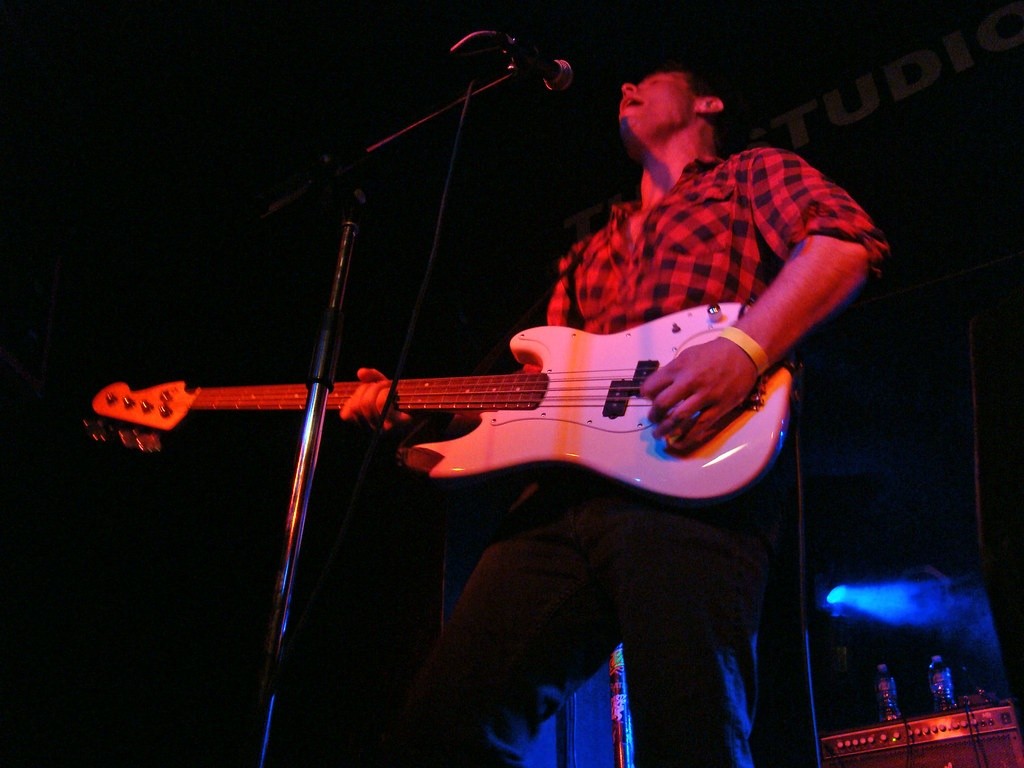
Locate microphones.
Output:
[495,31,572,91]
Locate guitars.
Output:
[82,301,793,501]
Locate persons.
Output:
[339,62,891,768]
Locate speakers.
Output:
[821,705,1024,768]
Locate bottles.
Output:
[928,656,956,712]
[874,664,901,723]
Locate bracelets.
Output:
[720,326,770,375]
